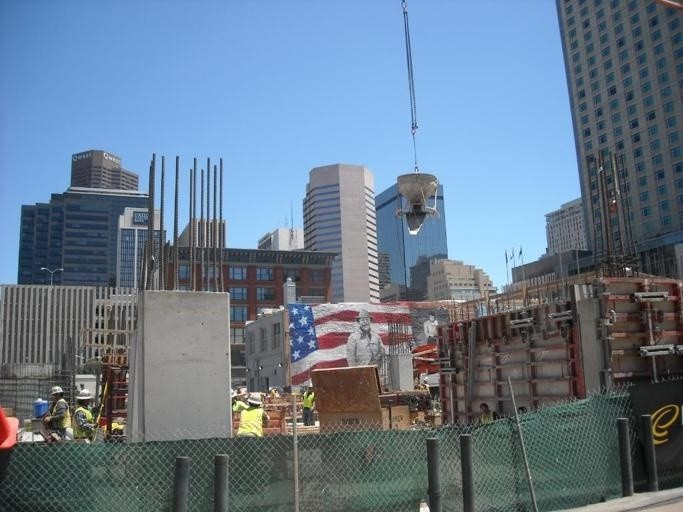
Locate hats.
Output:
[355,309,373,323]
[429,311,435,316]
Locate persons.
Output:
[423,311,438,344]
[302,383,315,426]
[346,309,386,367]
[41,386,104,442]
[232,388,270,439]
[476,403,498,423]
[409,395,425,424]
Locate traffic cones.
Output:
[0,405,21,452]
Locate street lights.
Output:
[39,266,64,286]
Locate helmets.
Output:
[231,389,238,398]
[77,390,93,400]
[247,393,263,404]
[50,386,64,396]
[308,383,314,389]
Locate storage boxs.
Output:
[33,398,51,417]
[310,365,410,483]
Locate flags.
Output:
[289,304,411,385]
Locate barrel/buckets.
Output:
[34,403,49,418]
